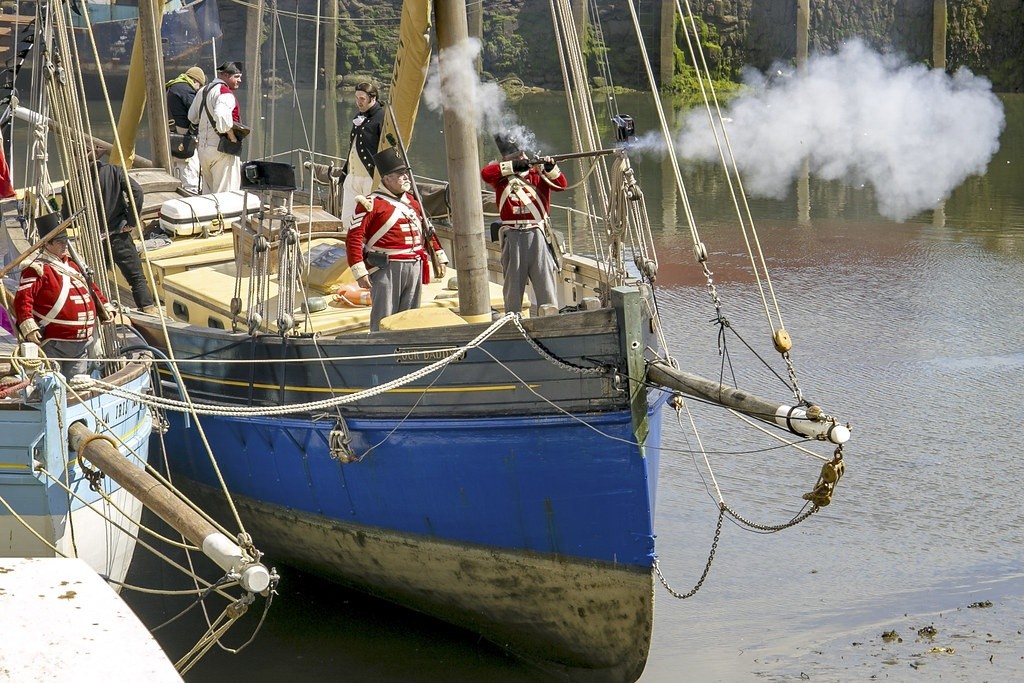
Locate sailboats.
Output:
[0,0,854,682]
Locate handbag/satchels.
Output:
[169,133,196,158]
[217,121,250,157]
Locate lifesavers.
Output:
[336,281,374,306]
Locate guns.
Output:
[385,97,444,279]
[530,146,627,166]
[37,190,111,325]
[211,35,218,79]
[0,204,88,279]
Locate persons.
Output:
[13,211,117,385]
[480,123,569,321]
[345,145,450,333]
[187,61,244,195]
[60,142,156,314]
[165,67,207,193]
[339,82,385,234]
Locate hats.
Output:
[74,142,108,161]
[35,212,71,241]
[216,61,243,74]
[373,146,412,179]
[494,126,527,160]
[186,66,206,86]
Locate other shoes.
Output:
[141,304,160,315]
